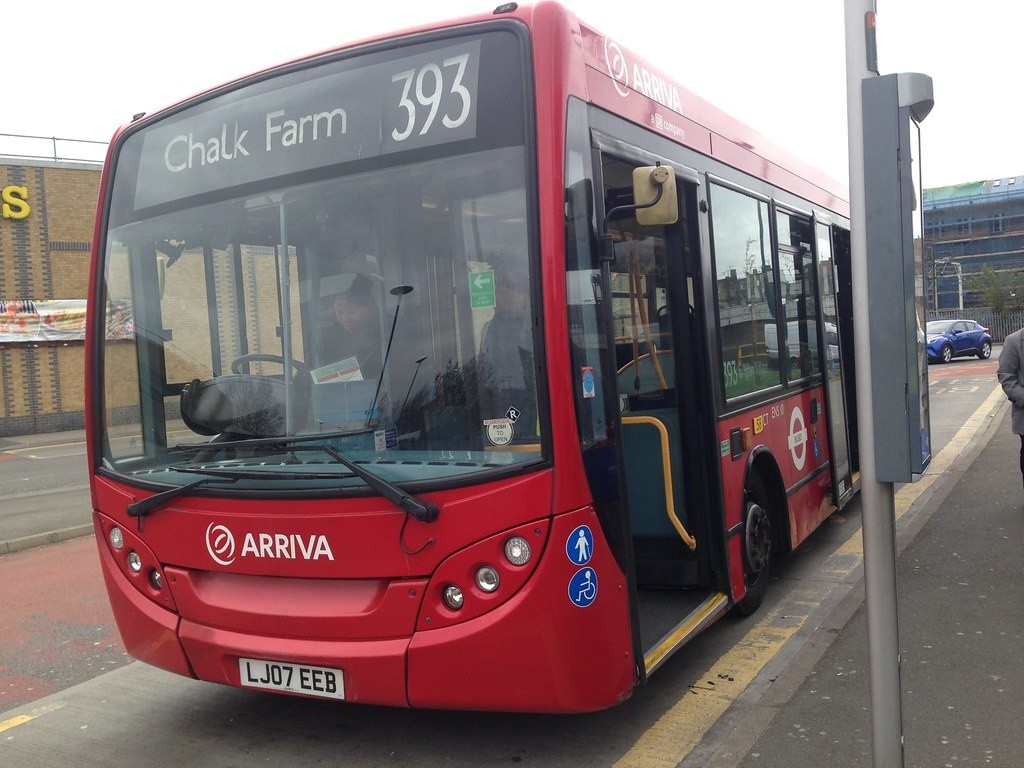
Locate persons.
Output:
[997,328,1024,486]
[295,273,538,442]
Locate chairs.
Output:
[615,299,694,409]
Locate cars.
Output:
[926,320,992,364]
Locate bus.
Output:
[85,1,857,714]
